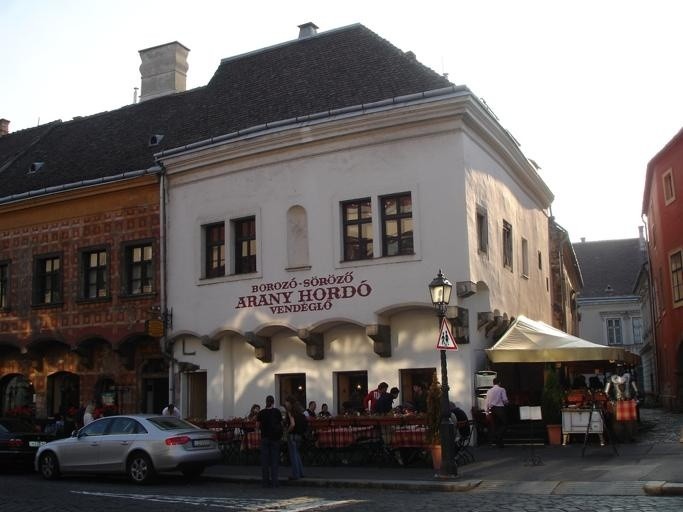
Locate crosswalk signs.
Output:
[436,317,458,351]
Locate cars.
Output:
[0,414,225,486]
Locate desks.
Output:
[319,426,374,463]
[248,431,263,448]
[452,420,478,465]
[392,425,432,448]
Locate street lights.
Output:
[429,269,458,481]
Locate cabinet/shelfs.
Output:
[560,408,604,447]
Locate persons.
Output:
[604,362,639,443]
[64,401,108,431]
[256,395,313,489]
[249,404,260,416]
[304,400,331,417]
[588,365,608,390]
[161,404,182,418]
[363,382,401,415]
[484,378,509,430]
[412,381,429,412]
[448,401,471,456]
